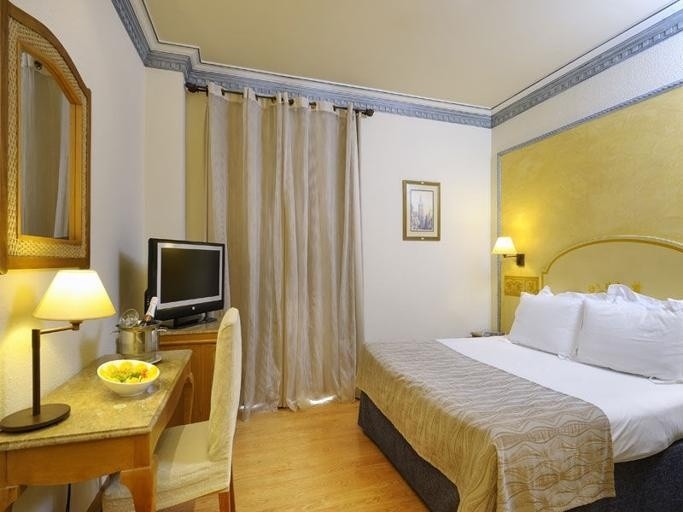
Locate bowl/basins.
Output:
[96,358,161,398]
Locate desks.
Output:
[1,349,196,512]
[158,316,221,428]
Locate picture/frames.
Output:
[402,180,441,240]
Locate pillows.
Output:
[508,282,680,385]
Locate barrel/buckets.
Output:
[115,319,169,363]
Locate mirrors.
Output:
[1,0,92,274]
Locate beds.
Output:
[358,234,682,510]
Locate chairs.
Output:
[98,307,243,512]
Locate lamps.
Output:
[491,236,524,267]
[0,268,117,433]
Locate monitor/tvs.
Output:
[144,238,225,330]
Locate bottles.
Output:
[138,296,159,326]
